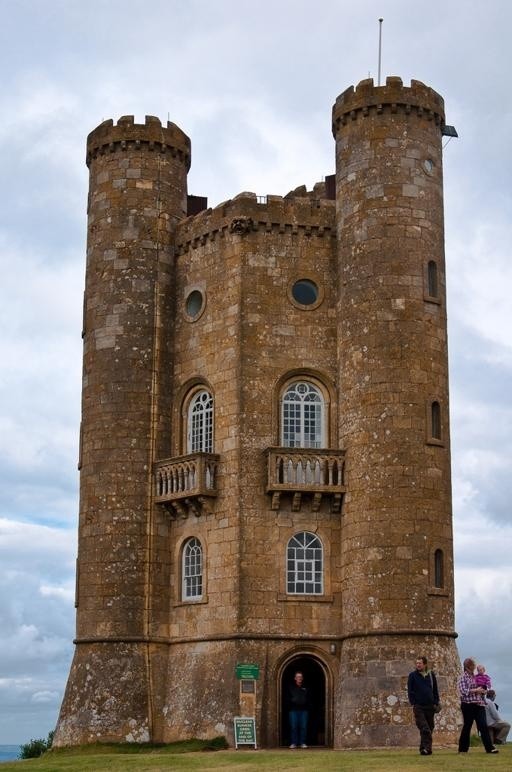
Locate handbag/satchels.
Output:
[434,701,443,712]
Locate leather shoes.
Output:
[421,749,431,755]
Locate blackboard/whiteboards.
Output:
[233,718,257,744]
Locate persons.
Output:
[458,658,499,753]
[407,656,438,755]
[283,669,313,749]
[471,664,492,705]
[485,690,511,744]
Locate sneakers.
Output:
[290,743,297,748]
[301,743,307,749]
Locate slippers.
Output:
[487,748,498,754]
